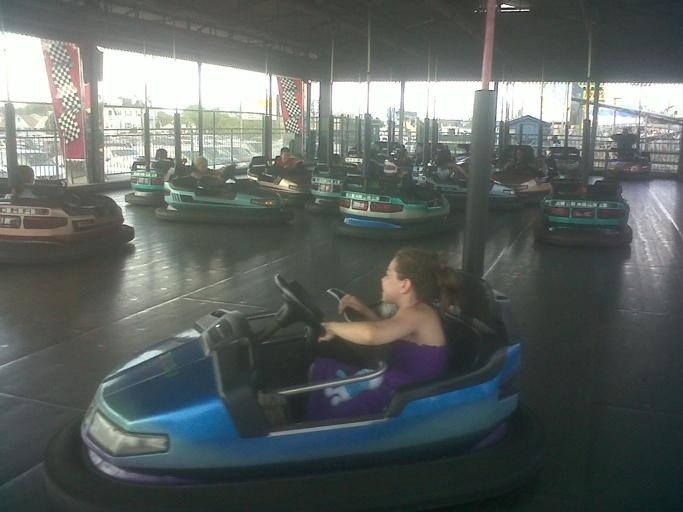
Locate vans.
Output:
[404,141,416,152]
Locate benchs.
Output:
[364,270,485,372]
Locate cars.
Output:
[140,132,262,169]
[0,131,140,149]
[0,149,65,180]
[104,147,139,175]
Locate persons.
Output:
[151,148,174,170]
[303,241,465,418]
[504,147,528,170]
[190,157,209,179]
[274,147,295,168]
[18,165,39,199]
